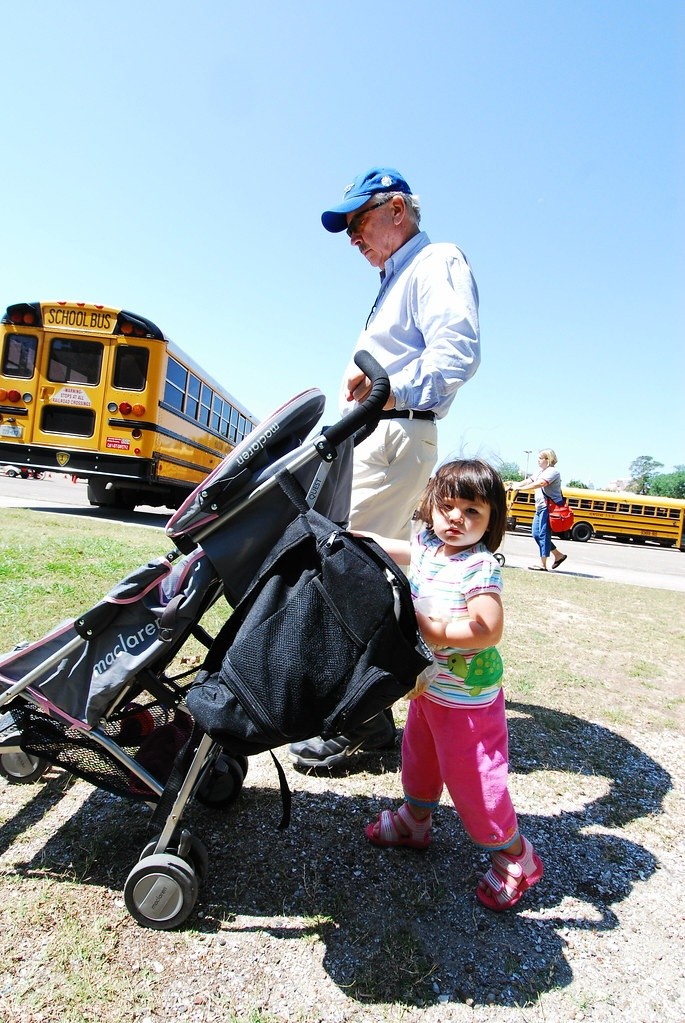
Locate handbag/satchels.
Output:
[188,510,435,756]
[544,497,574,532]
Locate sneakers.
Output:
[288,707,397,766]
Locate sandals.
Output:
[477,834,543,910]
[365,802,432,846]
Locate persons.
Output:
[288,166,480,767]
[349,455,543,911]
[511,448,567,571]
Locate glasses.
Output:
[346,195,394,239]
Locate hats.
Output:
[321,166,413,233]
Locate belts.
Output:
[380,410,435,422]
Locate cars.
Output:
[0,464,89,484]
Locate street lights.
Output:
[523,450,532,480]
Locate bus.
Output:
[411,475,434,525]
[0,300,263,514]
[502,480,685,553]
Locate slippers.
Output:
[528,565,548,571]
[552,555,567,569]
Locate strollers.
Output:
[0,349,393,932]
[490,488,520,567]
